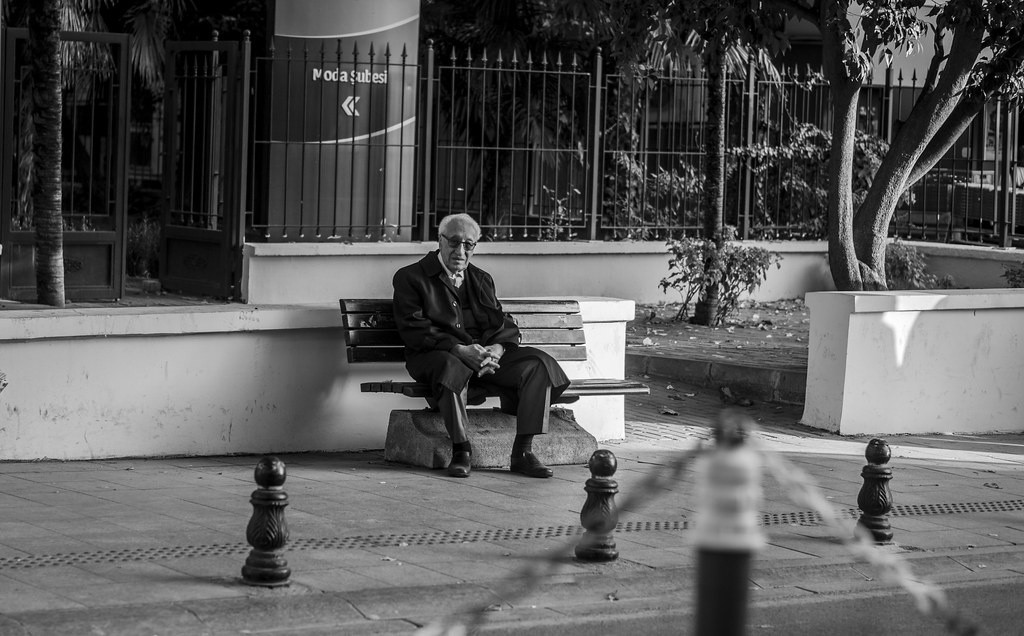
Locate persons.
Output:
[393,213,570,477]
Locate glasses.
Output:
[442,234,477,251]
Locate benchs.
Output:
[338,289,652,472]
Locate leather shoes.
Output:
[449,448,470,478]
[511,451,553,479]
